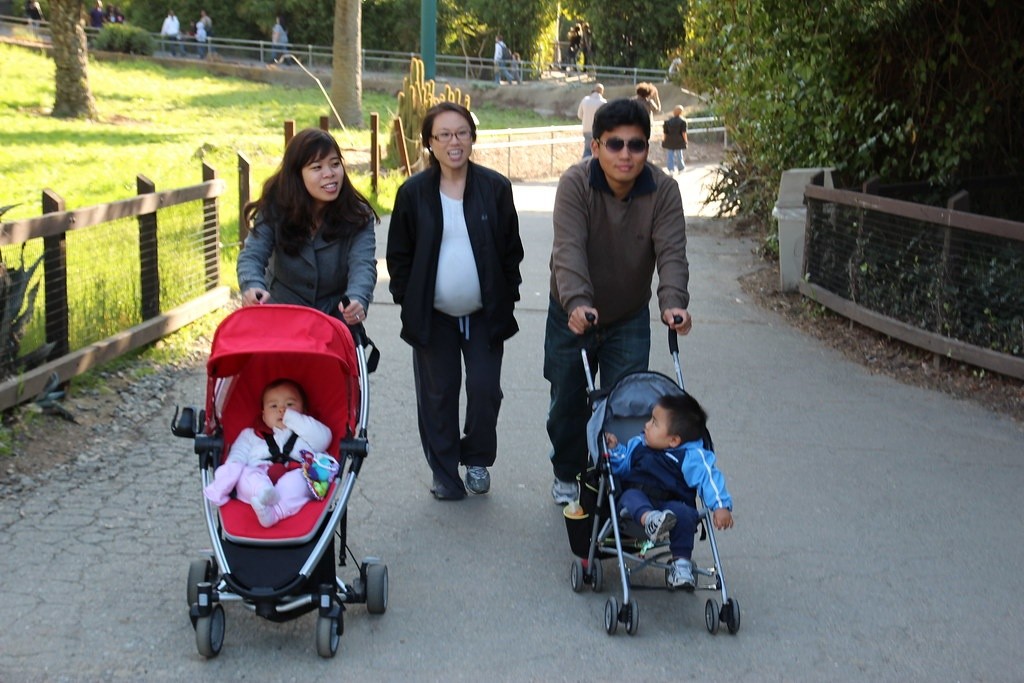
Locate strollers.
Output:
[171,293,389,659]
[564,312,740,635]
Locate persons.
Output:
[214,375,331,528]
[385,101,524,500]
[266,15,292,66]
[662,105,688,176]
[510,53,523,85]
[606,395,735,591]
[631,82,662,124]
[235,129,379,334]
[158,8,213,60]
[493,34,515,86]
[662,51,681,86]
[564,22,596,73]
[24,0,124,41]
[544,99,690,504]
[577,83,608,157]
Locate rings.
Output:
[356,314,360,320]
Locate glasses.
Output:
[430,130,472,142]
[595,137,648,152]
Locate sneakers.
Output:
[465,464,490,494]
[430,470,466,501]
[664,555,696,592]
[644,509,676,546]
[551,476,579,504]
